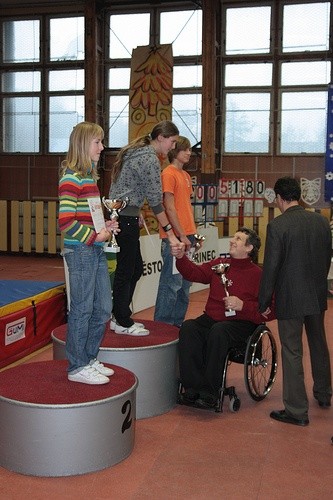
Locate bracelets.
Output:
[162,223,172,232]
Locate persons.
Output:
[58,122,121,385]
[169,229,277,409]
[258,176,333,426]
[109,121,184,336]
[154,136,198,327]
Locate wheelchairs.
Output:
[177,321,278,413]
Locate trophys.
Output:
[192,234,206,261]
[102,196,130,247]
[211,263,234,312]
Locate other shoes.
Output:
[178,393,199,403]
[195,398,219,407]
[314,394,331,408]
[270,410,309,426]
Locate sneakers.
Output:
[67,365,110,384]
[91,360,114,376]
[110,320,150,336]
